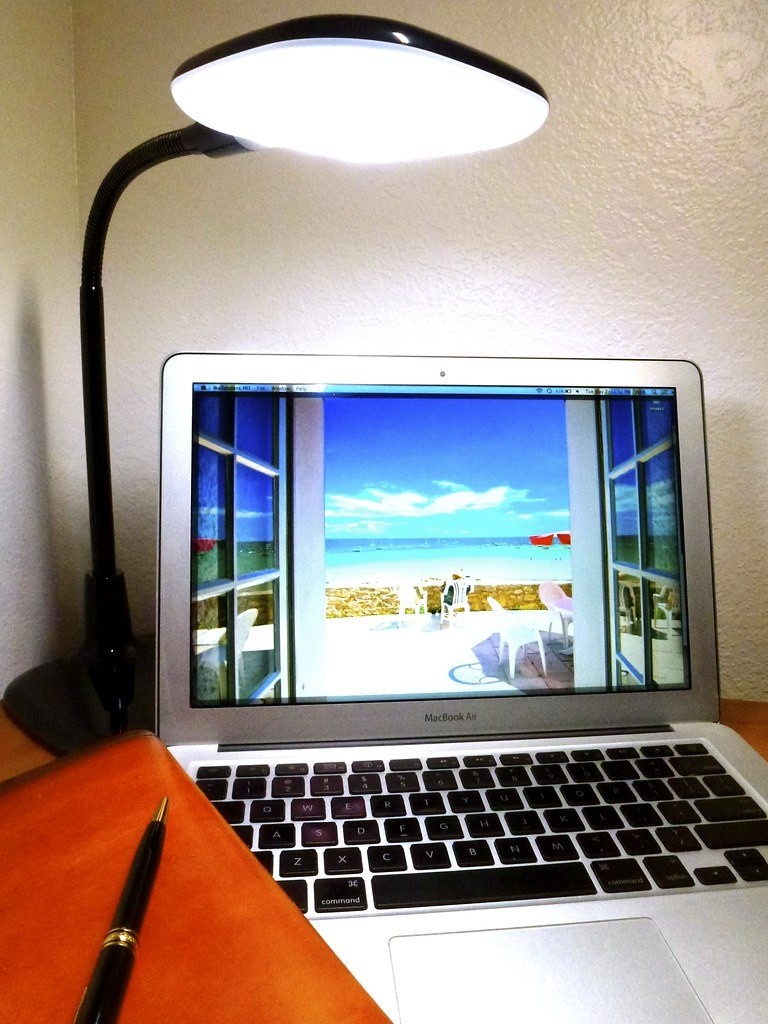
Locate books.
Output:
[0,731,394,1024]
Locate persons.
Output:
[440,572,470,614]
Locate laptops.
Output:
[155,351,768,1024]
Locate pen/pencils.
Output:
[72,794,169,1024]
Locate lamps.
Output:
[0,14,549,756]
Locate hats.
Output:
[452,569,462,578]
